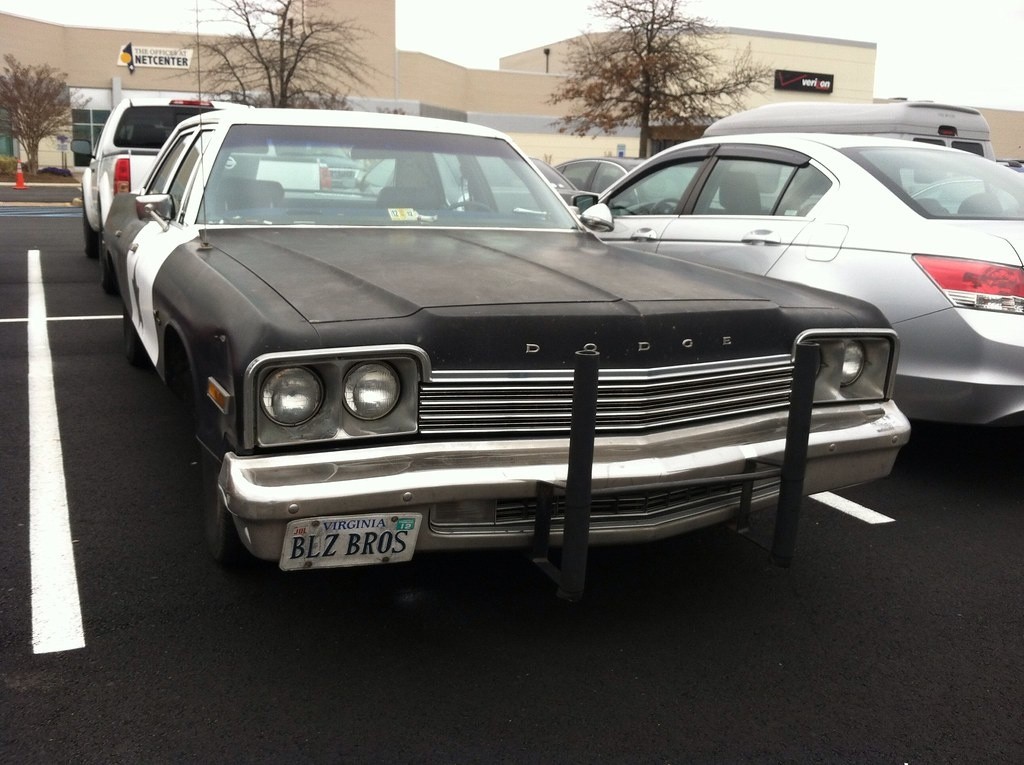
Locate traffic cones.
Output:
[12,160,28,190]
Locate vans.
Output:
[694,96,994,196]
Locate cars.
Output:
[101,103,911,575]
[579,133,1023,428]
[321,156,1024,270]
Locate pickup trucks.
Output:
[69,96,333,266]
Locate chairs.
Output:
[718,171,762,214]
[131,123,165,142]
[959,193,1004,214]
[375,156,434,210]
[213,177,285,213]
[916,199,950,217]
